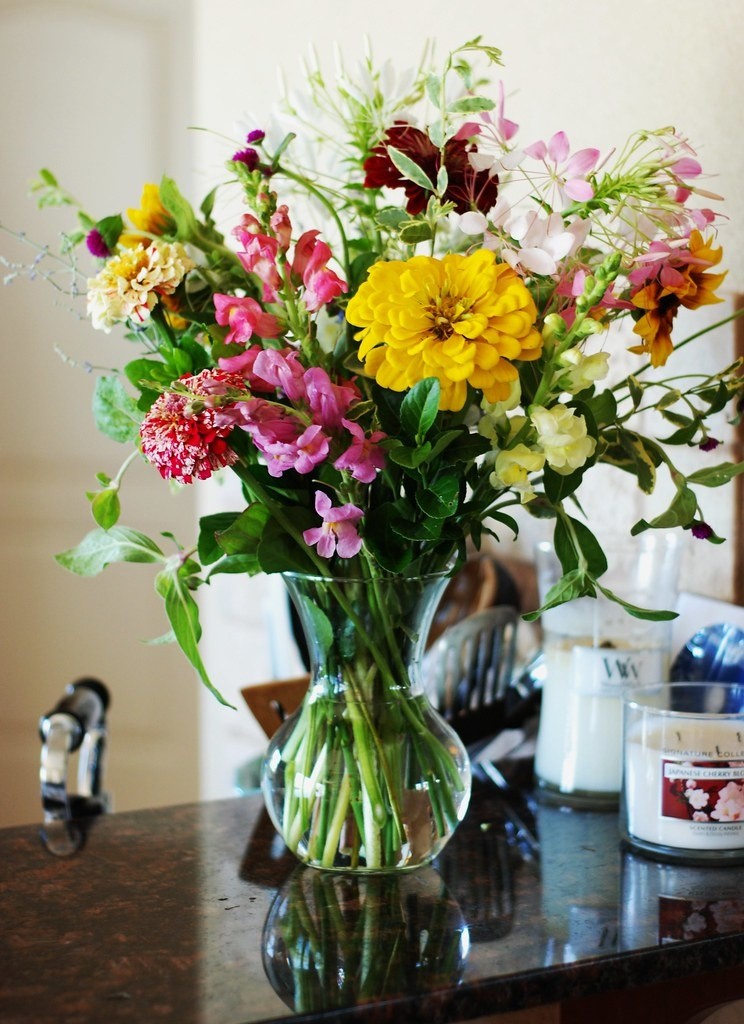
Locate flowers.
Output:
[0,26,743,869]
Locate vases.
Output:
[258,570,472,874]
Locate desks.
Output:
[1,785,744,1024]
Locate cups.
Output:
[618,682,744,864]
[524,534,687,811]
